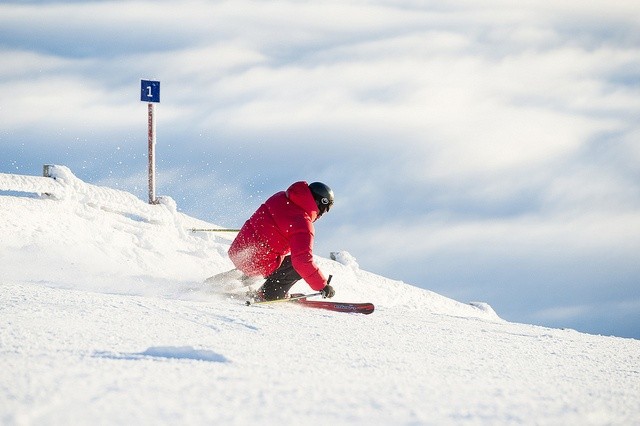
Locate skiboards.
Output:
[226,294,374,315]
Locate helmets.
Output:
[308,182,334,217]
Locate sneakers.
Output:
[256,288,291,302]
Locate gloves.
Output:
[320,285,336,299]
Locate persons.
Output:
[228,180,335,303]
